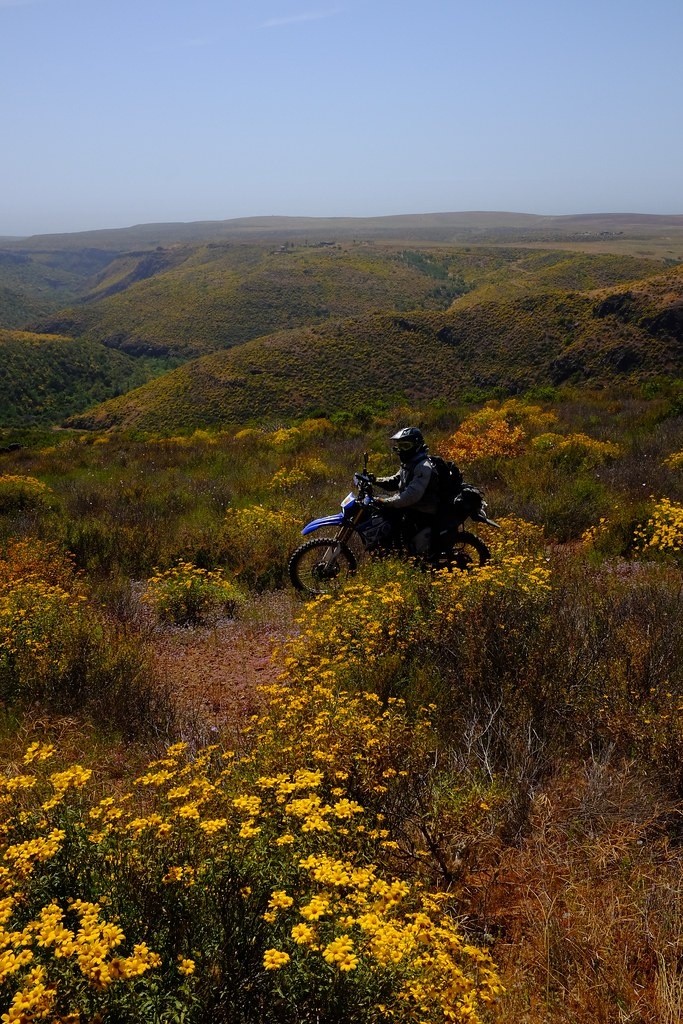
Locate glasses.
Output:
[396,442,419,450]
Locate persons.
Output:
[354,427,451,572]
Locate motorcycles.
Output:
[285,452,502,599]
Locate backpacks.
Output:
[429,456,463,503]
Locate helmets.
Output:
[390,427,429,463]
